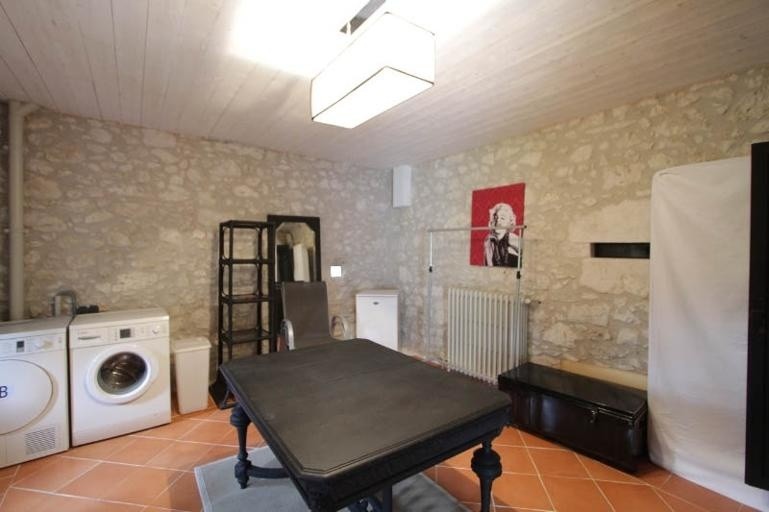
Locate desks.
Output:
[216,335,510,512]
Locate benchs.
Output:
[496,362,646,475]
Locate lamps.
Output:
[308,0,438,131]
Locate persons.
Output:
[279,231,295,283]
[483,203,521,266]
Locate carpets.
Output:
[192,442,470,512]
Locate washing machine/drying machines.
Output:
[68,306,172,447]
[0,316,73,469]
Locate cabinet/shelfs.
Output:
[213,212,278,410]
[354,288,401,353]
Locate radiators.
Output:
[447,284,532,389]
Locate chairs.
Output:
[275,281,348,349]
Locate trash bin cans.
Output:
[171,337,213,414]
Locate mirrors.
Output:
[268,213,321,353]
